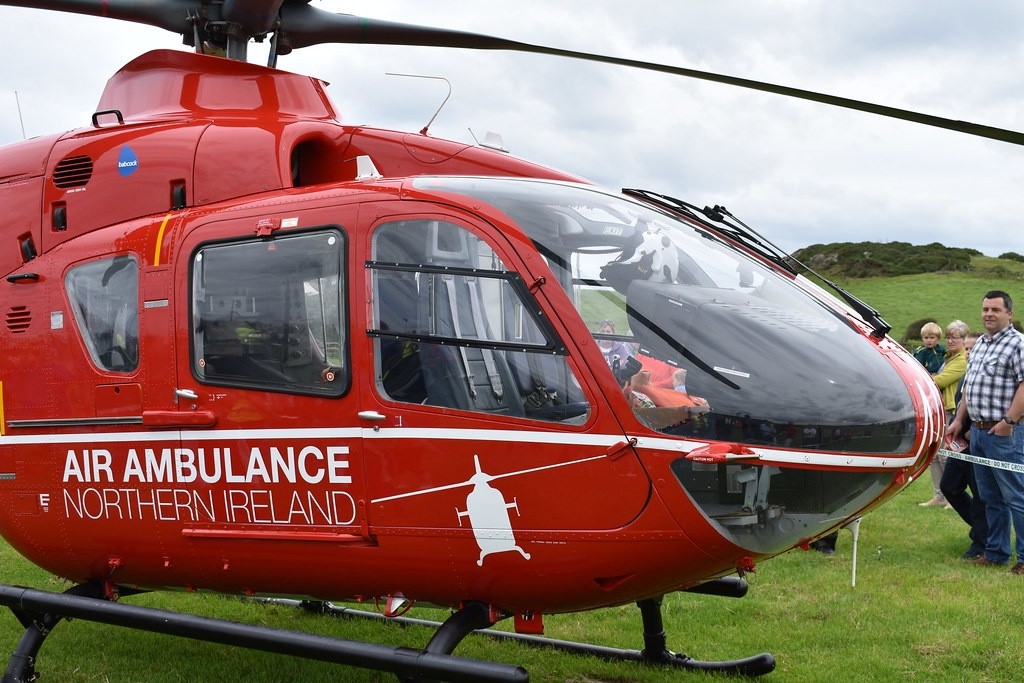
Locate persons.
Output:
[623,328,640,359]
[671,368,687,391]
[743,414,902,450]
[698,411,708,437]
[910,290,1024,575]
[595,320,628,372]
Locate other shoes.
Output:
[919,497,945,506]
[943,503,954,509]
[962,550,984,558]
[1007,562,1024,575]
[970,556,993,566]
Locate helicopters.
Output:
[0,0,1024,683]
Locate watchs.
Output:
[1001,416,1016,425]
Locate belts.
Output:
[974,416,1024,429]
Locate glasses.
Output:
[944,336,961,342]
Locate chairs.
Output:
[111,262,138,367]
[416,219,589,418]
[204,311,297,381]
